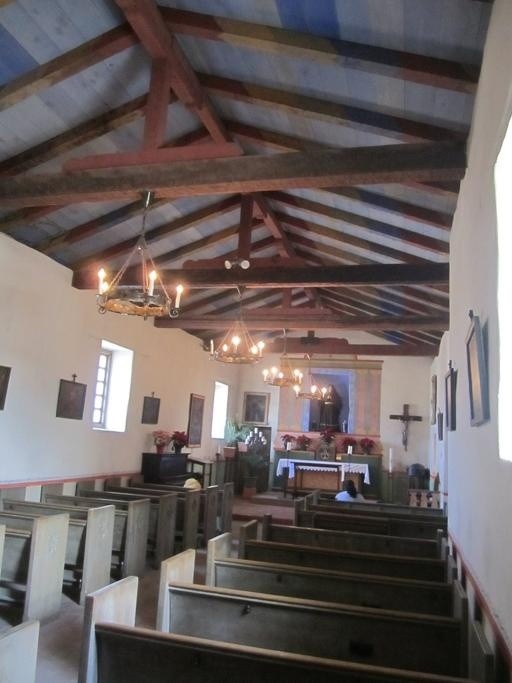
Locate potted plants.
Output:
[223,419,241,458]
[236,425,252,453]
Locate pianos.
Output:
[142,453,206,492]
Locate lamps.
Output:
[210,287,265,364]
[262,327,303,386]
[237,255,251,270]
[294,354,326,401]
[96,191,183,320]
[224,257,237,271]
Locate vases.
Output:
[175,447,181,453]
[156,446,164,454]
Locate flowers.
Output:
[170,431,187,448]
[280,427,374,455]
[152,431,173,446]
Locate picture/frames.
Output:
[464,311,489,426]
[431,375,437,425]
[444,359,455,431]
[186,393,205,448]
[438,408,443,440]
[242,391,271,426]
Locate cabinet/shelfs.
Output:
[141,453,191,482]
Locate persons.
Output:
[317,383,343,431]
[334,479,366,502]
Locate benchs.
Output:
[0,482,500,683]
